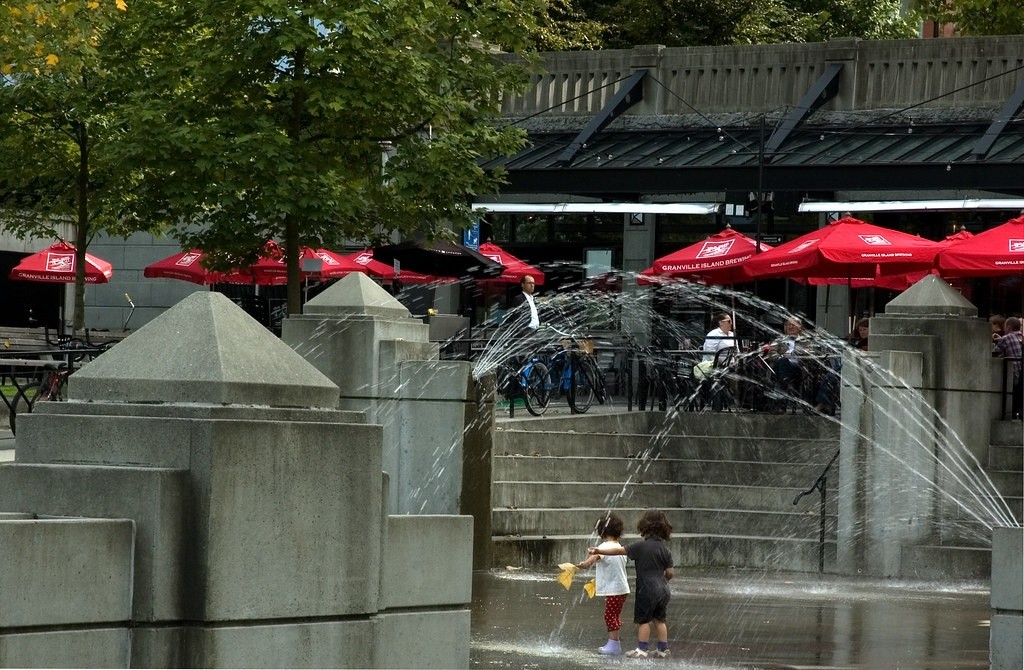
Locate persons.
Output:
[580,513,630,654]
[588,511,674,657]
[702,313,740,410]
[759,317,810,415]
[990,315,1024,384]
[803,319,869,414]
[515,273,540,399]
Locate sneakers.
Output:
[650,648,671,659]
[625,648,650,659]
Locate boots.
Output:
[598,639,622,655]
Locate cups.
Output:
[750,341,758,353]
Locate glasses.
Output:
[721,320,730,324]
[525,282,535,285]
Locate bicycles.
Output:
[520,337,598,417]
[9,292,136,437]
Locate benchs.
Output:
[0,326,134,395]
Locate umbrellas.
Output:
[144,232,544,358]
[637,209,1024,336]
[11,238,112,331]
